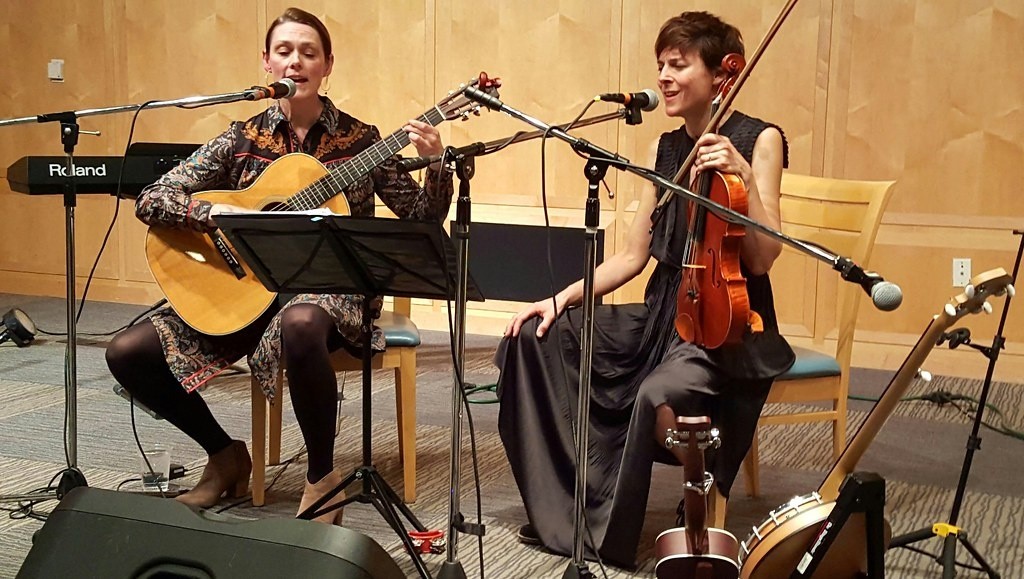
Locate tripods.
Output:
[211,214,481,579]
[888,228,1024,579]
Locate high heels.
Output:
[170,439,254,506]
[296,469,346,529]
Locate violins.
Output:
[674,51,757,354]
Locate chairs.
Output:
[711,172,898,530]
[251,297,419,506]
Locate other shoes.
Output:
[517,523,544,544]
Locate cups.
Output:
[139,444,173,492]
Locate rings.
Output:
[709,152,712,160]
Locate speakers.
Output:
[14,487,408,579]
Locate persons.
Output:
[106,7,456,524]
[489,11,797,574]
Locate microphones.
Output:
[245,78,297,102]
[594,89,660,112]
[846,258,903,312]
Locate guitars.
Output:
[651,413,741,579]
[141,70,503,340]
[737,266,1017,579]
